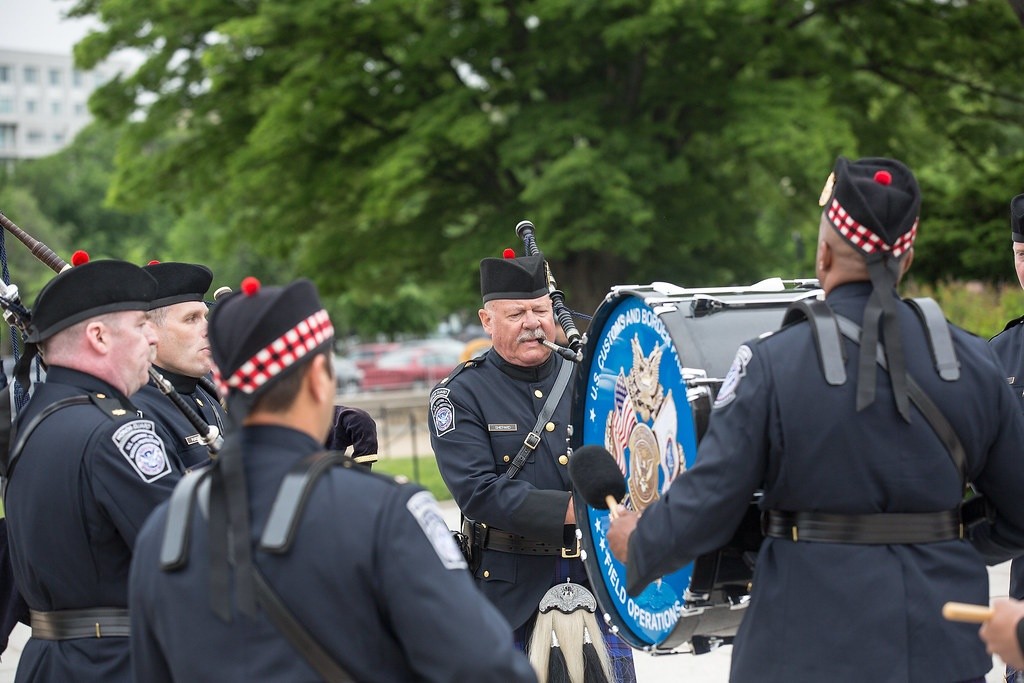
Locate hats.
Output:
[24,251,159,346]
[1010,193,1024,243]
[818,154,921,265]
[139,259,214,311]
[480,249,551,303]
[208,277,335,398]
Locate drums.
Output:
[567,278,827,655]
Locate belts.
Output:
[765,510,963,544]
[461,518,582,557]
[28,607,132,640]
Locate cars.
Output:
[334,336,490,395]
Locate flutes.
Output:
[1,213,234,461]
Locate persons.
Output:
[0,251,379,683]
[129,278,541,682]
[430,248,635,683]
[607,157,1008,683]
[978,192,1024,683]
